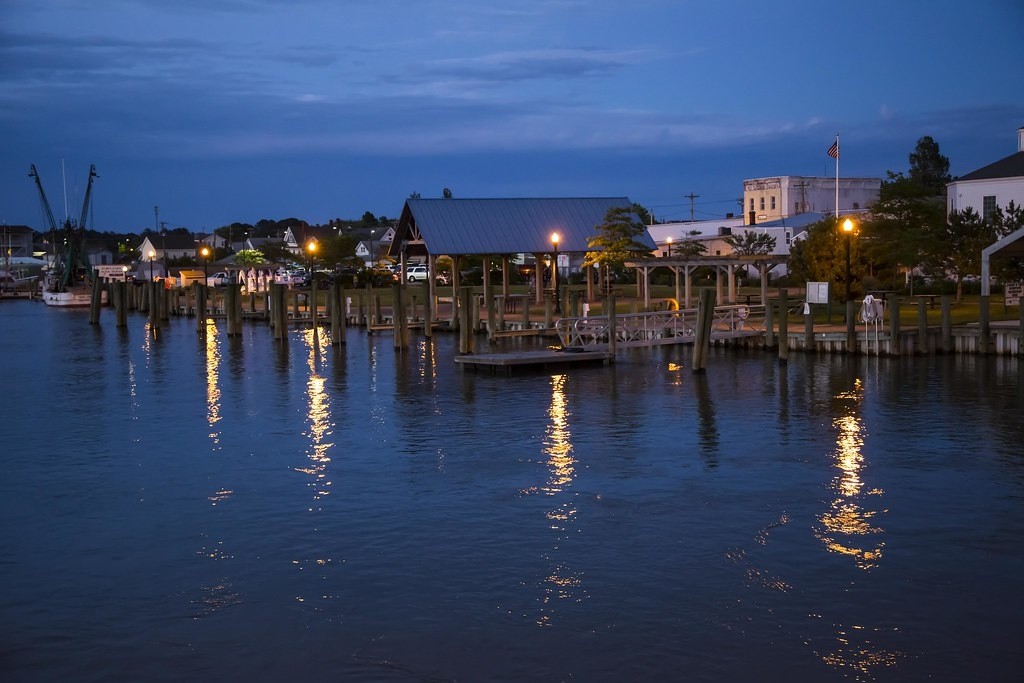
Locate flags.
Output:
[827,140,840,160]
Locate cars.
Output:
[127,260,552,288]
[568,263,617,286]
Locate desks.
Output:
[869,290,896,306]
[738,293,760,306]
[493,294,531,314]
[915,295,941,309]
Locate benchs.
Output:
[855,298,903,303]
[737,301,762,303]
[910,302,956,306]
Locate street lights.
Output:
[308,242,316,263]
[843,217,857,327]
[551,232,562,314]
[201,248,210,299]
[666,235,673,287]
[149,251,155,285]
[370,229,376,285]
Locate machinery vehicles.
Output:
[28,161,100,292]
